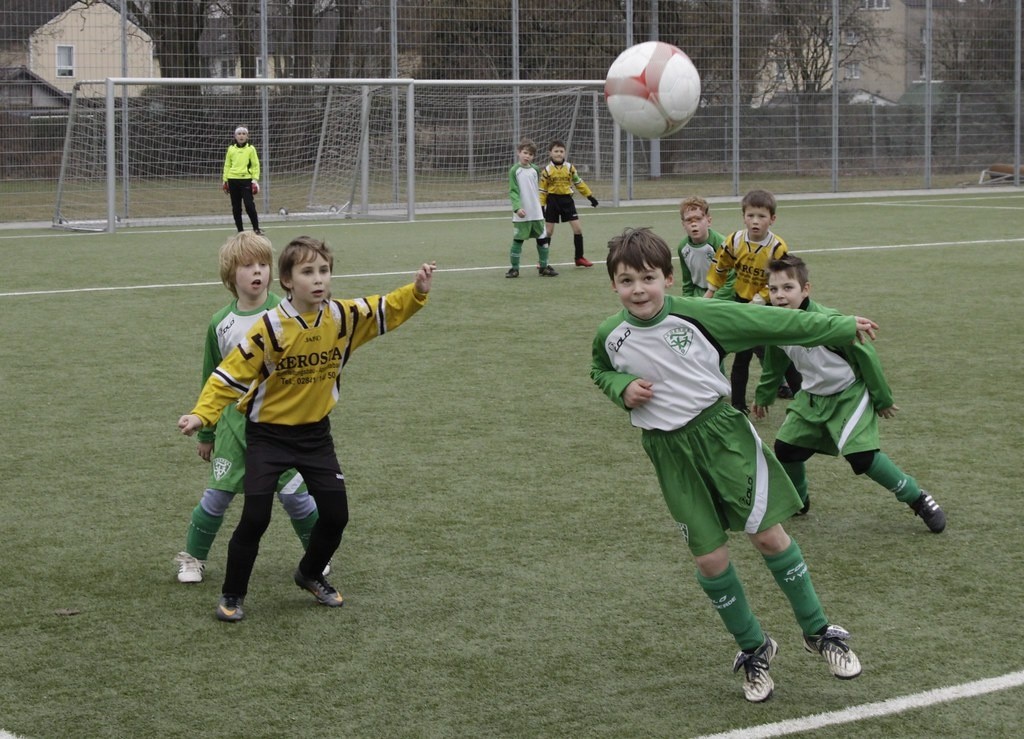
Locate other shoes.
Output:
[777,386,793,398]
[734,405,750,416]
[253,229,263,235]
[576,257,593,267]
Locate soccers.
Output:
[605,41,700,139]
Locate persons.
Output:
[537,141,599,268]
[678,197,793,399]
[177,235,437,621]
[222,125,263,236]
[703,189,802,418]
[752,251,946,535]
[505,139,559,279]
[589,227,880,702]
[178,230,330,583]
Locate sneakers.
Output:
[540,268,559,276]
[506,269,519,278]
[295,570,343,607]
[321,558,333,576]
[217,594,244,621]
[911,488,946,534]
[794,495,811,515]
[732,632,778,702]
[174,551,204,582]
[804,624,862,679]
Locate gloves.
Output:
[588,195,598,207]
[223,182,230,194]
[251,181,258,194]
